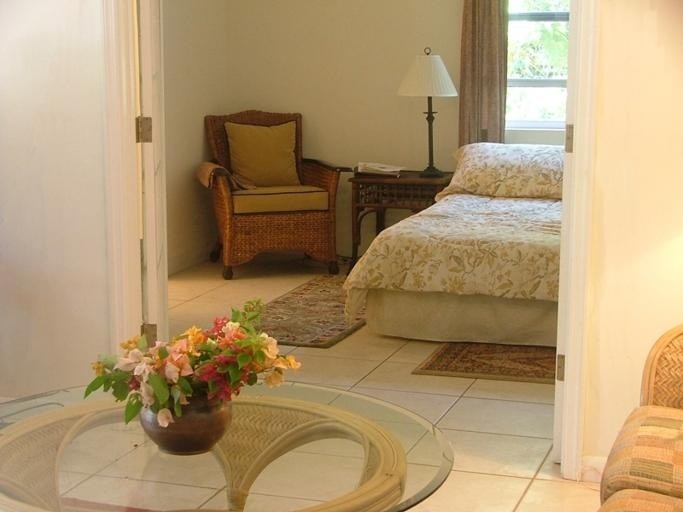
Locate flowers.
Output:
[88,292,303,431]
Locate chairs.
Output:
[199,106,352,279]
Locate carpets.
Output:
[410,340,557,381]
[255,268,370,348]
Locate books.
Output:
[354,162,400,176]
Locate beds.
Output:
[348,191,583,349]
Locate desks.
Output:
[346,168,455,276]
[0,379,454,510]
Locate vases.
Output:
[141,397,233,458]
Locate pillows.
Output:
[223,119,303,189]
[434,142,566,202]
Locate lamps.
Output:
[398,46,460,179]
